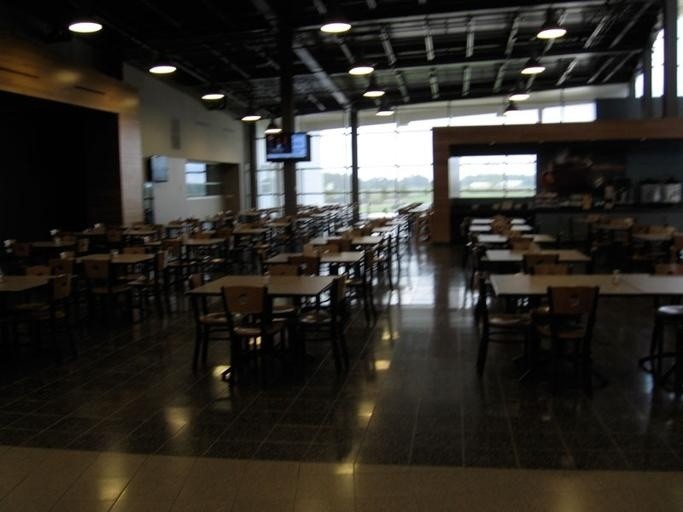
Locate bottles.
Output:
[612,269,620,286]
[263,270,273,291]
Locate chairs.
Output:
[466,218,683,432]
[2,201,422,398]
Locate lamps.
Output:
[521,49,546,76]
[318,1,396,117]
[535,6,567,40]
[67,8,284,134]
[504,92,517,112]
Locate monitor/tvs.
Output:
[266,132,310,162]
[150,155,169,182]
